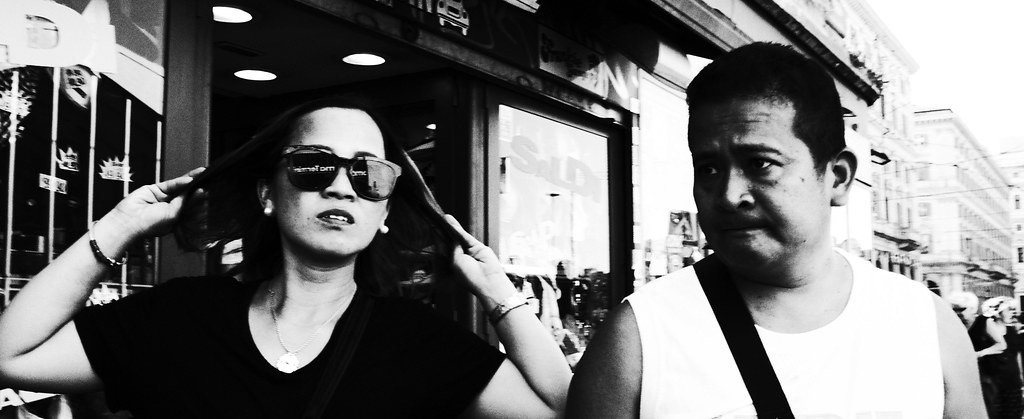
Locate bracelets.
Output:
[88,220,127,266]
[488,294,529,327]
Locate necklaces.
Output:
[264,273,352,373]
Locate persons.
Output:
[951,291,1024,419]
[564,41,988,418]
[0,83,576,419]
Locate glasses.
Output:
[278,143,406,203]
